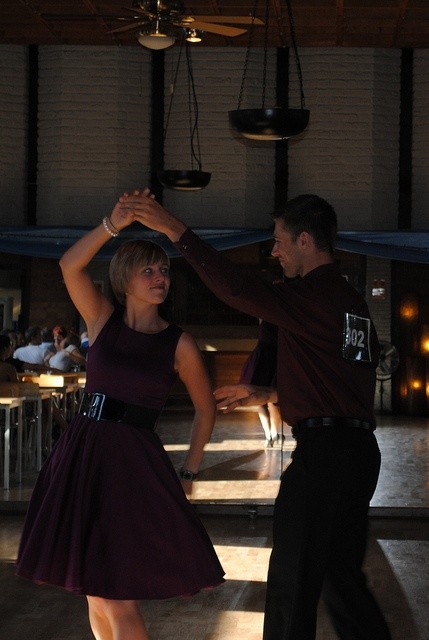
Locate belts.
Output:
[77,391,158,429]
[291,416,377,433]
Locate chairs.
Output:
[23,364,45,372]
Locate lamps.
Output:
[228,0,311,141]
[185,28,202,43]
[138,19,171,49]
[158,32,212,191]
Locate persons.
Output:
[117,189,395,640]
[0,327,90,433]
[240,312,284,449]
[14,189,225,640]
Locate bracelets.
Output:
[103,216,120,237]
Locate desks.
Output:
[0,372,87,490]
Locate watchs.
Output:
[178,467,198,480]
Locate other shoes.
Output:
[265,434,280,448]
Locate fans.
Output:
[374,341,402,416]
[42,1,264,36]
